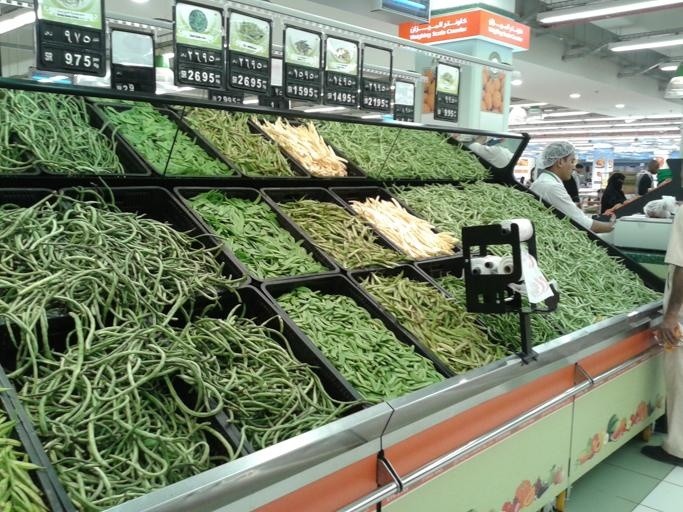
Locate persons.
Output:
[510,140,682,235]
[641,191,683,469]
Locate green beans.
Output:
[1,85,663,512]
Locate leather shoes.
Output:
[640,446,683,467]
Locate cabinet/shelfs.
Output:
[99,292,683,511]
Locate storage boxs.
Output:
[1,182,664,512]
[1,89,494,183]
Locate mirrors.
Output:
[1,86,526,188]
